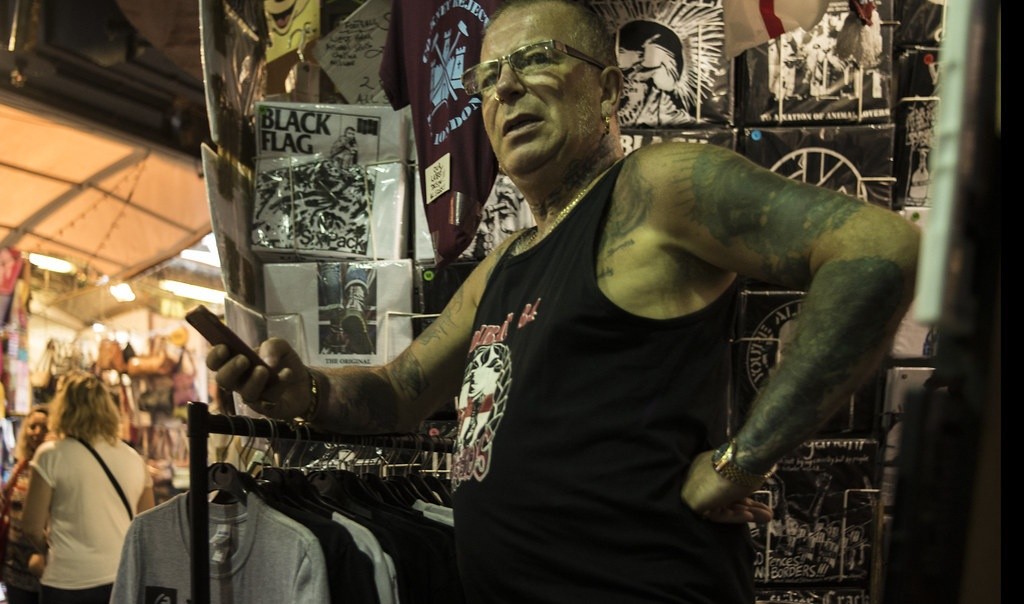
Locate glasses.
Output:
[459,39,606,96]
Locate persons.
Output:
[192,0,920,604]
[0,370,158,604]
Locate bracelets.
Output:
[285,363,321,430]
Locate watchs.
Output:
[713,436,779,489]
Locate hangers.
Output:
[204,414,450,508]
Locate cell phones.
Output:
[185,304,280,392]
